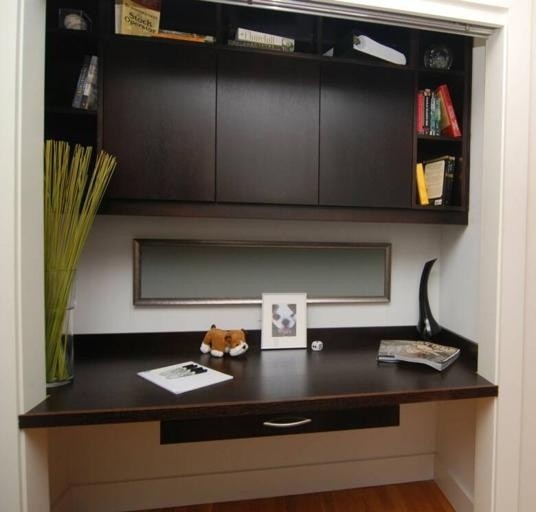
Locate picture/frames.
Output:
[261,293,307,350]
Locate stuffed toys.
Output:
[200,324,250,358]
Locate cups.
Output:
[45,268,77,388]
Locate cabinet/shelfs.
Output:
[45,1,476,225]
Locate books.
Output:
[229,27,295,52]
[418,84,462,140]
[417,156,456,207]
[396,339,461,372]
[115,0,161,36]
[377,339,401,364]
[161,30,216,45]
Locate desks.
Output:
[18,324,497,512]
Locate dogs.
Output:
[272,304,296,337]
